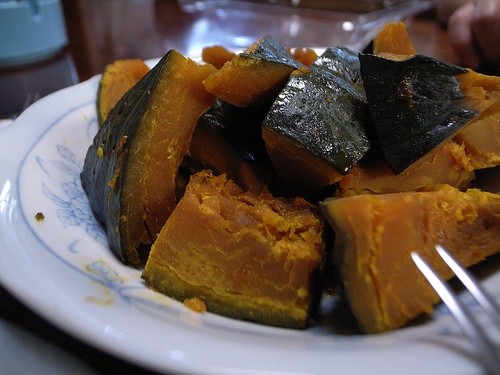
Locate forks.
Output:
[411,245,500,375]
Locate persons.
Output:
[436,0,500,74]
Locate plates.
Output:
[0,47,500,375]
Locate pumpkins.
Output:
[78,19,500,335]
